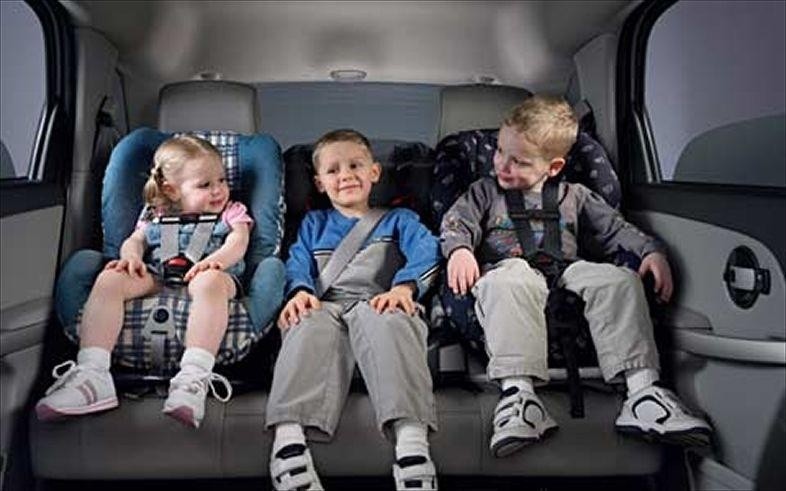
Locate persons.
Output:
[264,127,445,490]
[35,131,254,428]
[437,91,713,459]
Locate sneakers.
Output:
[390,462,440,491]
[268,450,327,491]
[34,365,120,423]
[160,366,215,429]
[489,387,561,460]
[613,385,716,450]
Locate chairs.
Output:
[27,80,662,480]
